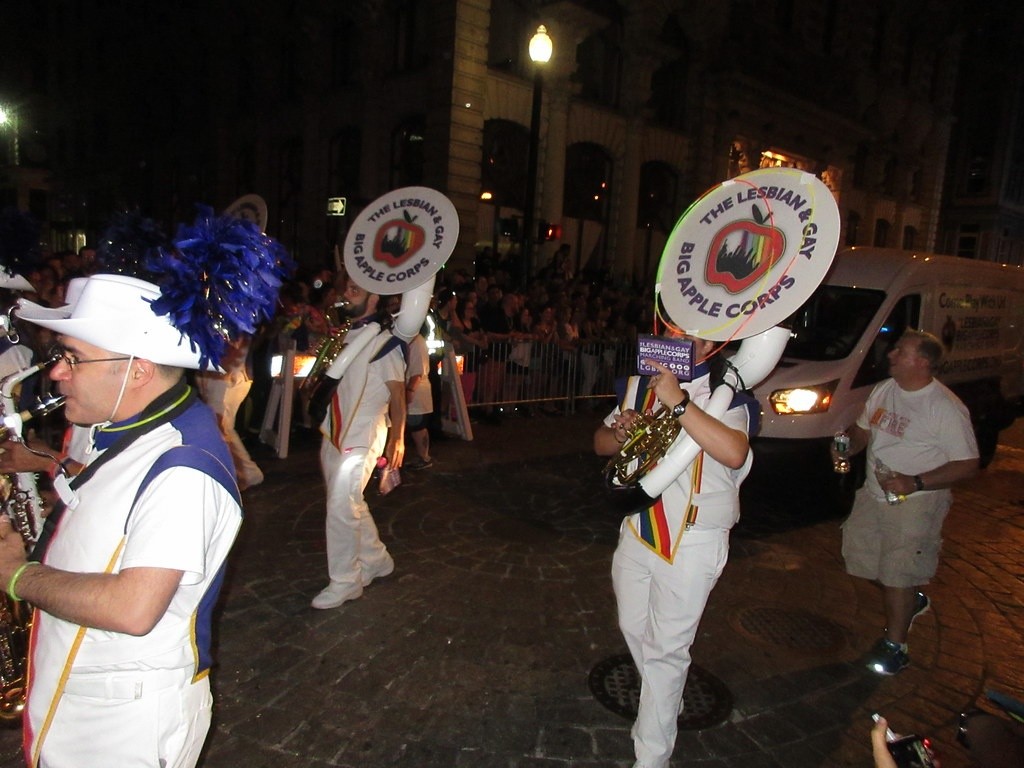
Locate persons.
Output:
[1,192,811,540]
[0,270,248,767]
[307,270,408,612]
[829,327,981,680]
[593,312,757,763]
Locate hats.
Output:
[0,264,37,294]
[15,219,288,374]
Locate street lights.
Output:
[525,23,556,291]
[0,105,19,169]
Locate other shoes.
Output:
[468,402,614,423]
[630,696,683,740]
[238,466,264,492]
[633,759,676,768]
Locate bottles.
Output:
[834,426,850,473]
[874,459,900,504]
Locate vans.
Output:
[749,246,1024,470]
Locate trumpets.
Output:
[296,319,350,393]
[595,402,678,490]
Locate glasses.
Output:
[46,345,140,373]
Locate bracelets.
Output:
[614,428,626,444]
[913,475,924,491]
[9,560,41,602]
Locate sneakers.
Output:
[359,550,394,586]
[882,593,929,633]
[312,578,363,609]
[405,456,432,471]
[865,637,909,676]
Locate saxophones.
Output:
[0,390,75,720]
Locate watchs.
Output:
[672,388,690,419]
